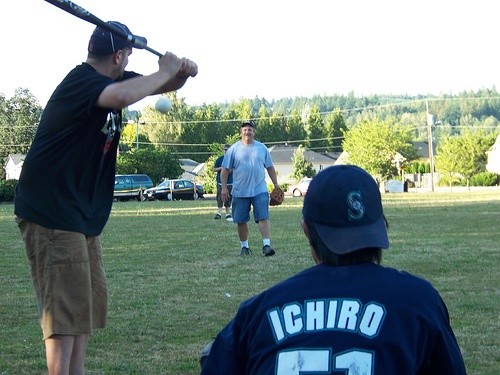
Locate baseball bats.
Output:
[44,0,198,77]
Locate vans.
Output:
[113,174,153,202]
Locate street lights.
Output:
[428,114,443,190]
[128,115,144,150]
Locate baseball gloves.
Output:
[269,188,285,206]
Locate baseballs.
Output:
[155,98,171,112]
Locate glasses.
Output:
[224,149,228,151]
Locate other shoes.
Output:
[262,245,275,256]
[240,246,252,255]
[215,214,221,219]
[226,215,231,220]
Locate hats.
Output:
[88,21,147,55]
[241,121,254,128]
[302,165,390,255]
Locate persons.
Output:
[213,145,233,219]
[199,165,468,375]
[14,21,198,375]
[220,122,284,256]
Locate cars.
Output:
[288,178,313,197]
[143,179,205,201]
